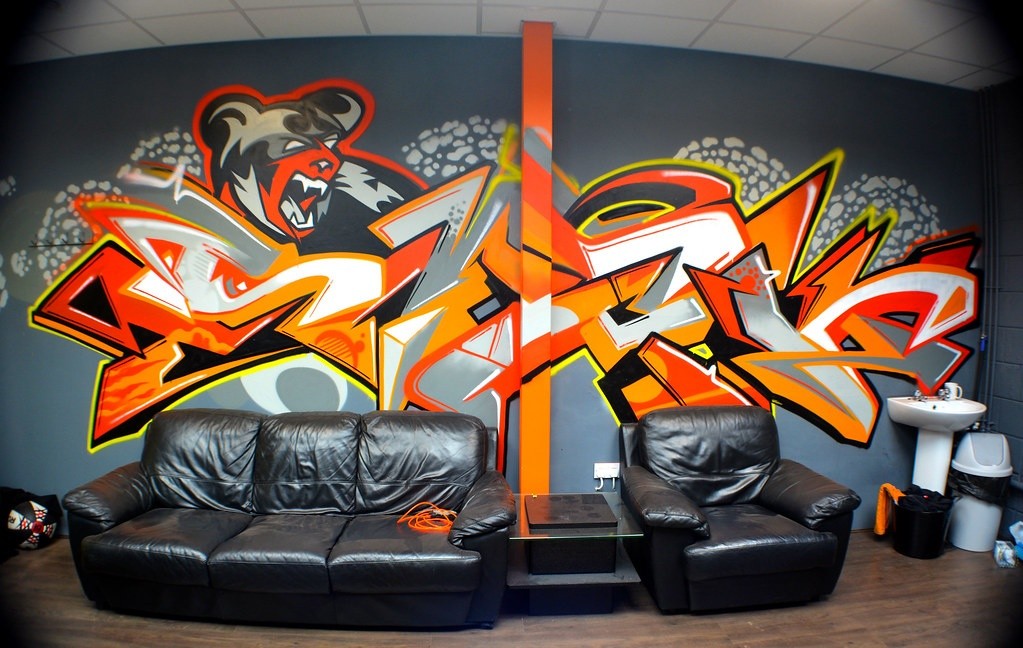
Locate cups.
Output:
[944,382,963,400]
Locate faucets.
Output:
[914,389,922,401]
[938,388,949,400]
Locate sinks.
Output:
[886,396,987,432]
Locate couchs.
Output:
[619,406,862,615]
[60,409,517,632]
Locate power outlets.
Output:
[594,463,620,478]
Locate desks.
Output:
[504,491,644,615]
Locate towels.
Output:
[874,482,906,535]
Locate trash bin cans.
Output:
[946,432,1014,554]
